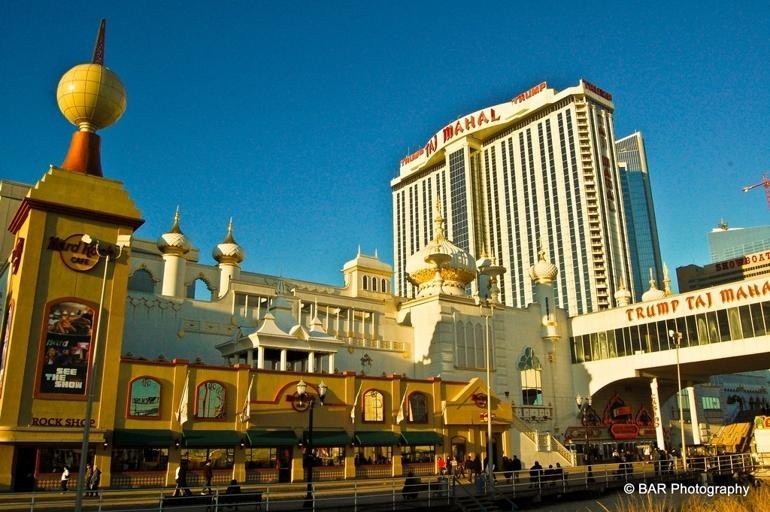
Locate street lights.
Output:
[73,230,136,511]
[576,391,597,489]
[668,329,689,474]
[293,376,328,508]
[471,286,499,502]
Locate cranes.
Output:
[739,175,770,219]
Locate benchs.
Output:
[536,472,569,489]
[405,481,443,498]
[613,470,635,480]
[159,490,268,512]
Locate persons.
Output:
[84,464,93,498]
[226,479,242,510]
[586,466,596,484]
[41,309,94,368]
[59,464,70,494]
[171,460,192,495]
[436,455,567,487]
[91,466,102,497]
[201,458,215,495]
[610,447,740,476]
